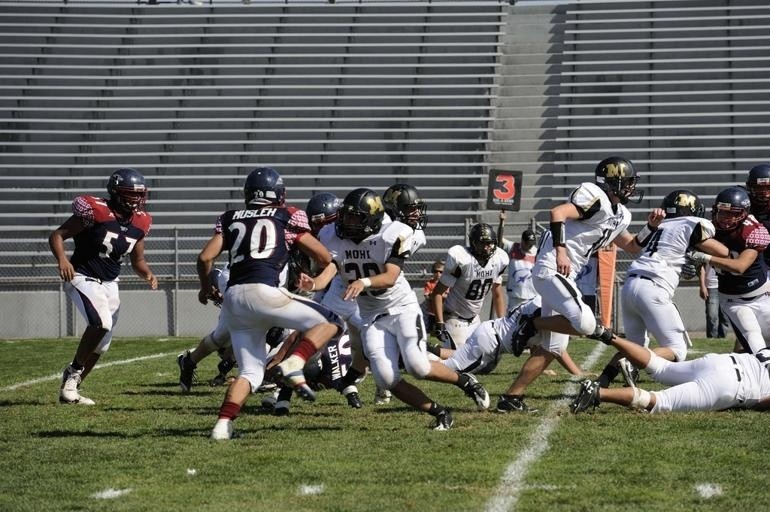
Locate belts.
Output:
[730,355,740,381]
[737,296,756,301]
[628,273,651,279]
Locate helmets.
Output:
[244,168,429,240]
[660,164,770,235]
[107,168,147,212]
[468,223,497,260]
[210,268,226,286]
[595,157,644,205]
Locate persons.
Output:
[48,167,159,406]
[176,156,770,438]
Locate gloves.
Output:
[681,262,700,280]
[686,250,711,265]
[436,322,452,345]
[199,287,217,304]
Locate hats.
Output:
[522,229,536,245]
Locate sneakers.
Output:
[211,420,235,440]
[569,379,599,415]
[588,325,615,344]
[495,393,537,415]
[432,411,454,433]
[619,356,636,388]
[512,310,542,358]
[61,363,95,404]
[459,372,491,411]
[179,350,392,414]
